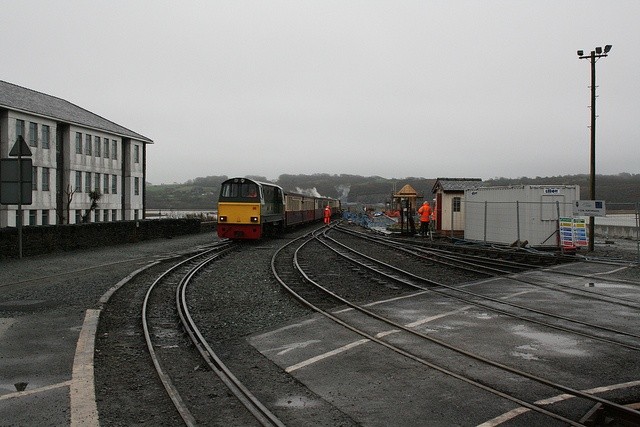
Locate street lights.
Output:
[577,45,612,251]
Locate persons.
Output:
[324,205,331,224]
[418,200,432,237]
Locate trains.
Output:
[217,177,343,244]
